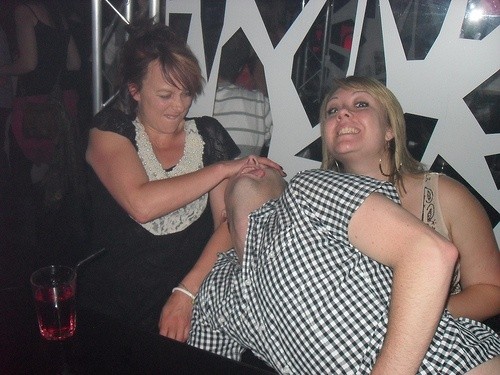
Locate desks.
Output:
[1,292,274,375]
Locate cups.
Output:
[30,265,80,340]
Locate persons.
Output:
[2,64,75,177]
[319,77,500,333]
[185,154,500,375]
[213,49,274,158]
[3,0,80,90]
[78,20,286,343]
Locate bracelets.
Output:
[173,287,196,303]
